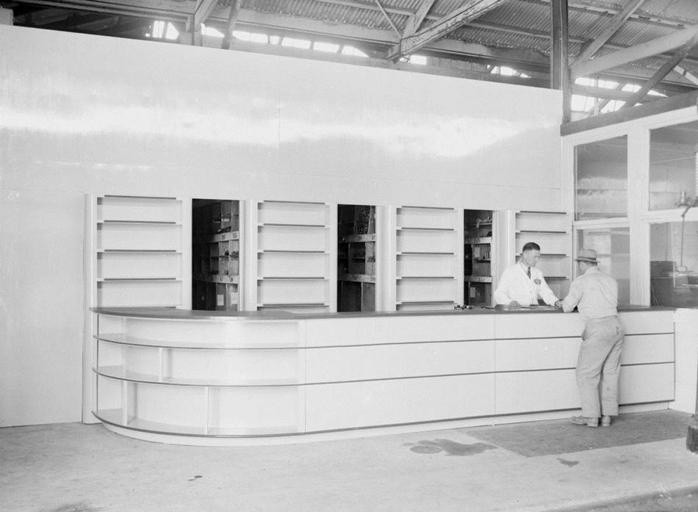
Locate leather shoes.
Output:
[570,414,611,427]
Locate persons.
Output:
[494,242,559,309]
[559,248,624,427]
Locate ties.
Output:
[526,266,532,280]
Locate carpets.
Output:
[466,410,697,457]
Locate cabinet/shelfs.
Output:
[191,199,241,313]
[466,208,492,309]
[650,262,698,309]
[384,202,464,307]
[511,209,574,298]
[337,206,377,312]
[247,198,333,308]
[83,193,188,306]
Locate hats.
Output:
[575,248,598,263]
